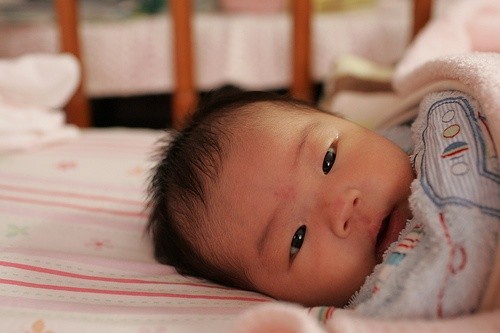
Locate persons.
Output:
[142,49,500,321]
